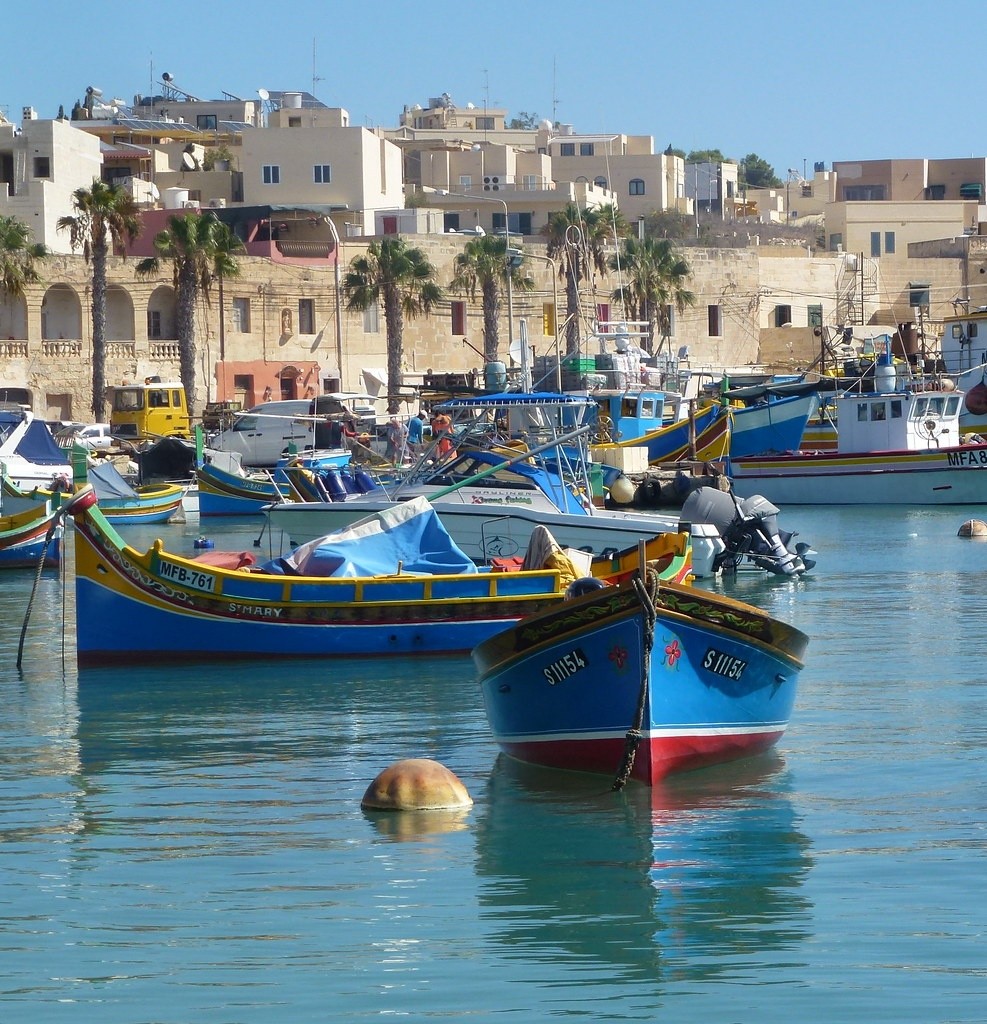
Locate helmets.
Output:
[564,577,603,601]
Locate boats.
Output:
[0,141,986,569]
[66,440,696,670]
[472,539,810,787]
[260,471,819,579]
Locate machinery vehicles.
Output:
[108,379,191,441]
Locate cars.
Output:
[45,420,120,450]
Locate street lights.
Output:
[436,190,514,380]
[507,248,561,394]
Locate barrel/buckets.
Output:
[874,363,896,392]
[483,361,507,390]
[844,360,859,377]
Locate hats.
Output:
[420,410,428,420]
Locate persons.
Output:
[387,418,409,465]
[406,410,428,463]
[432,411,458,459]
